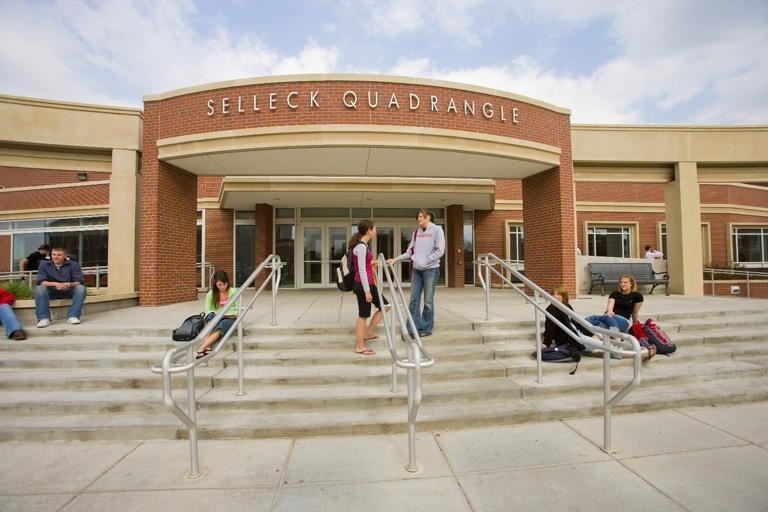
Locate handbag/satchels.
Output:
[643,318,676,354]
[533,346,581,365]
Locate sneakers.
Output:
[36,318,52,327]
[66,317,79,324]
[9,329,26,340]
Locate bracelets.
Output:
[67,282,71,288]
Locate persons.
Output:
[0,288,26,341]
[347,219,393,356]
[541,287,656,365]
[385,207,446,342]
[193,270,240,359]
[644,245,664,259]
[585,273,644,343]
[34,245,87,328]
[17,243,50,277]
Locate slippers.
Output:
[196,347,212,358]
[364,335,378,341]
[361,349,375,355]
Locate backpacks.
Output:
[335,241,368,293]
[173,311,205,341]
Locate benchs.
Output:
[587,260,672,295]
